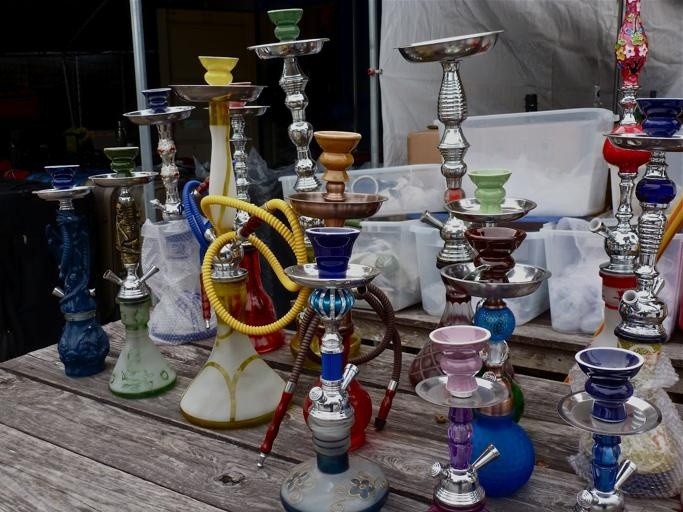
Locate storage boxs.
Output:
[412,225,548,328]
[278,163,450,215]
[406,129,440,164]
[435,107,616,217]
[345,220,421,312]
[539,222,682,342]
[608,153,683,222]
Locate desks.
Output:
[2,296,681,510]
